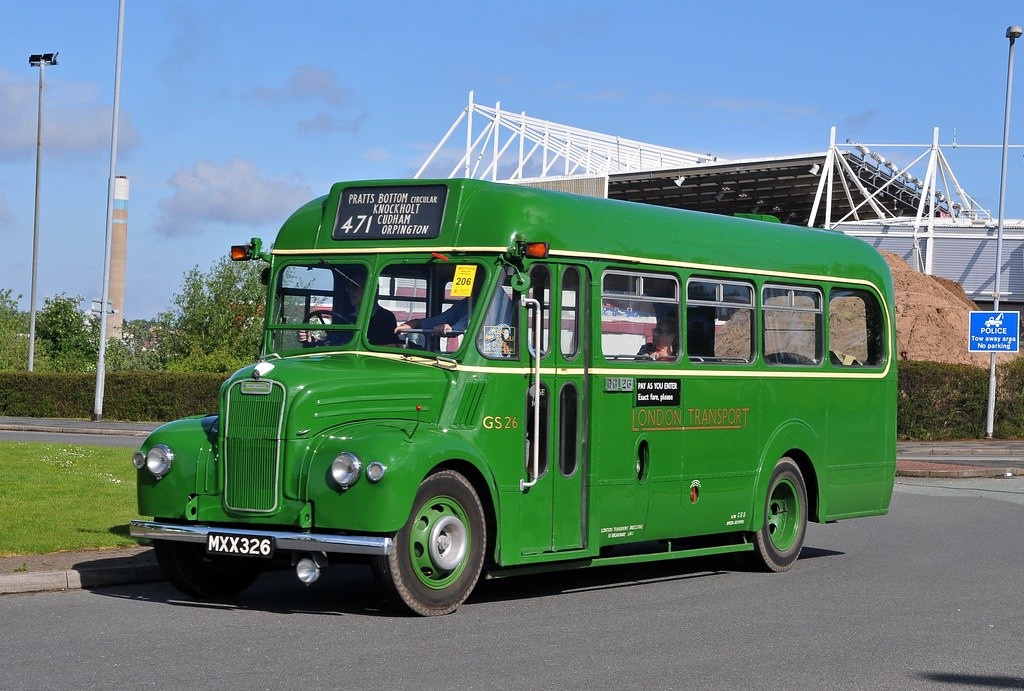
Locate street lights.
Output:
[25,52,60,368]
[982,25,1023,439]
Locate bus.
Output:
[128,175,901,615]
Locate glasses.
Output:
[345,283,364,292]
[652,328,669,335]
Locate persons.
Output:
[395,267,520,360]
[634,320,678,363]
[298,272,398,349]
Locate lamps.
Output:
[751,204,759,215]
[713,192,725,203]
[913,177,922,192]
[886,160,896,178]
[935,191,945,207]
[673,174,685,188]
[871,151,884,170]
[899,168,912,187]
[809,163,820,176]
[855,142,869,161]
